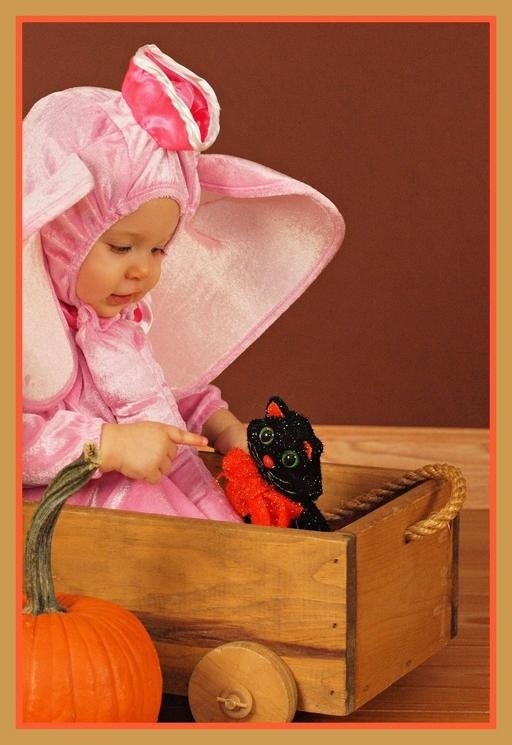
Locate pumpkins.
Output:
[22,444,166,723]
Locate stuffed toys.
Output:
[216,397,328,533]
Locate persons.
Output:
[21,43,347,522]
